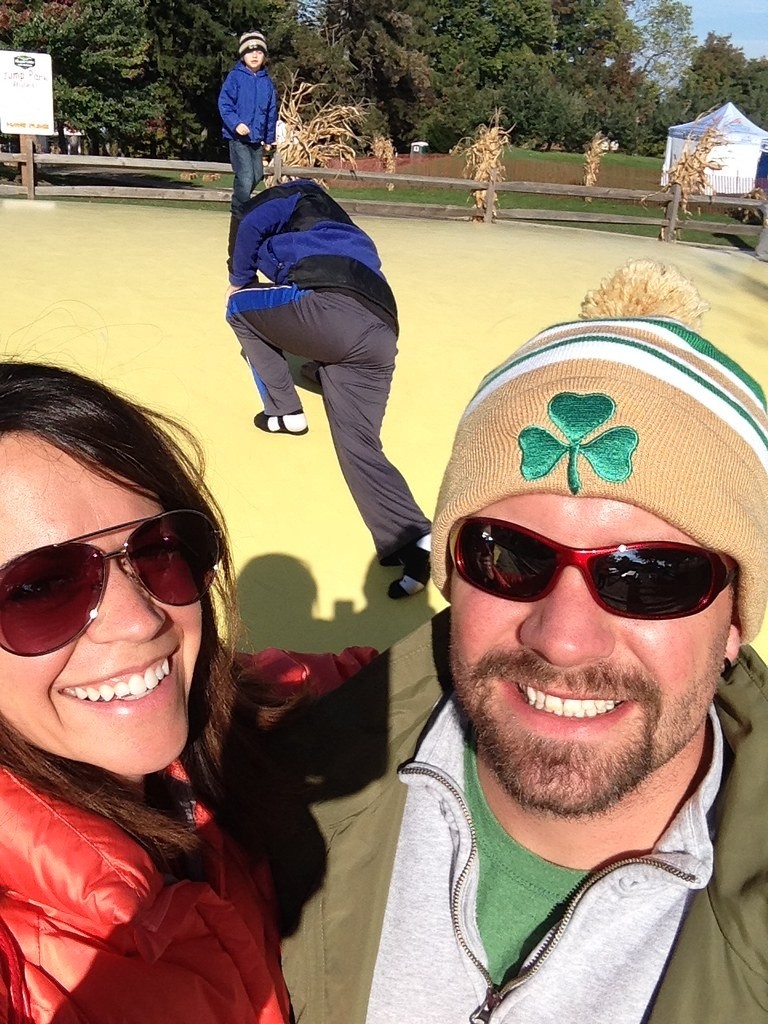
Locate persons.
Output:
[217,30,277,217]
[227,178,432,600]
[227,312,768,1024]
[0,360,382,1024]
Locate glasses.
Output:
[0,511,226,657]
[449,516,738,619]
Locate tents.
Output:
[661,102,768,195]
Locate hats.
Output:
[238,31,268,56]
